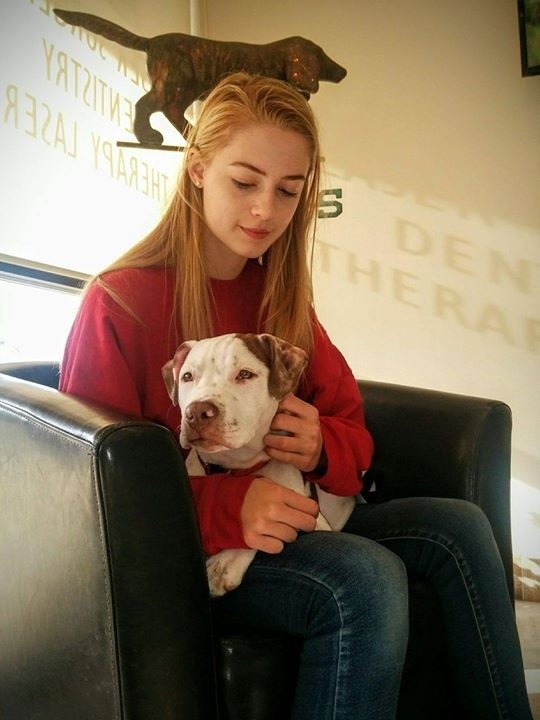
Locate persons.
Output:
[59,71,533,720]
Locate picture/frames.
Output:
[517,0,540,77]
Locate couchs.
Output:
[1,359,511,719]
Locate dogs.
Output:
[51,7,347,147]
[159,333,356,597]
